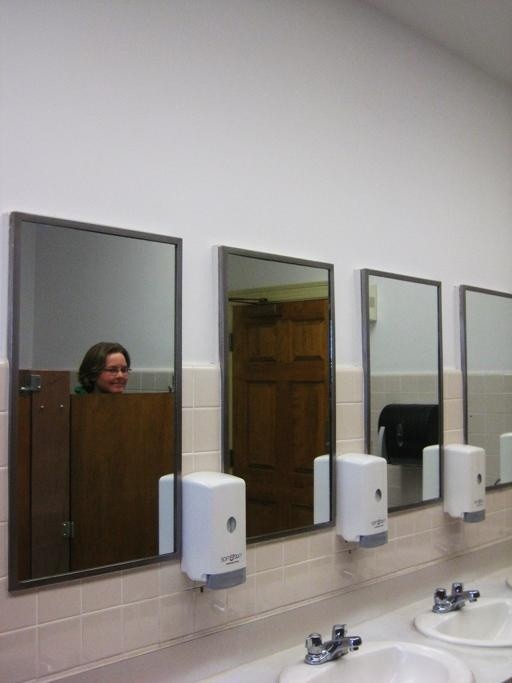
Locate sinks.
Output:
[278,639,475,683]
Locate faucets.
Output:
[432,581,480,614]
[302,623,364,664]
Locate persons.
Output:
[76,341,133,395]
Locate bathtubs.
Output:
[414,595,512,646]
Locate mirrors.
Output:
[458,285,512,491]
[360,268,444,514]
[217,246,336,546]
[8,212,181,592]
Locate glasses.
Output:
[102,366,133,376]
